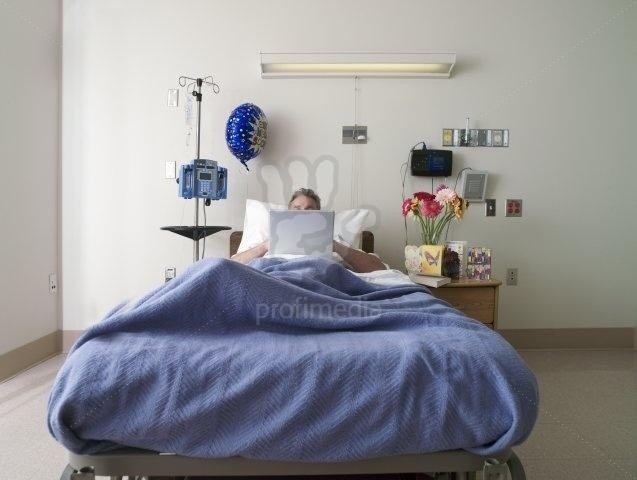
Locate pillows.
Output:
[236,198,378,264]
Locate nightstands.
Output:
[405,271,503,338]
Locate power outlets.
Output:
[48,273,57,294]
[165,267,174,284]
[506,268,518,287]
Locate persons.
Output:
[227,186,388,274]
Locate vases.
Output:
[419,243,445,278]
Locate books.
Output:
[408,240,491,289]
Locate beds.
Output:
[46,230,538,480]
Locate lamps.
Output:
[258,50,457,145]
[444,166,488,242]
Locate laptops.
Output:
[269,209,336,256]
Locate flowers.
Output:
[400,184,467,244]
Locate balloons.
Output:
[224,101,268,172]
